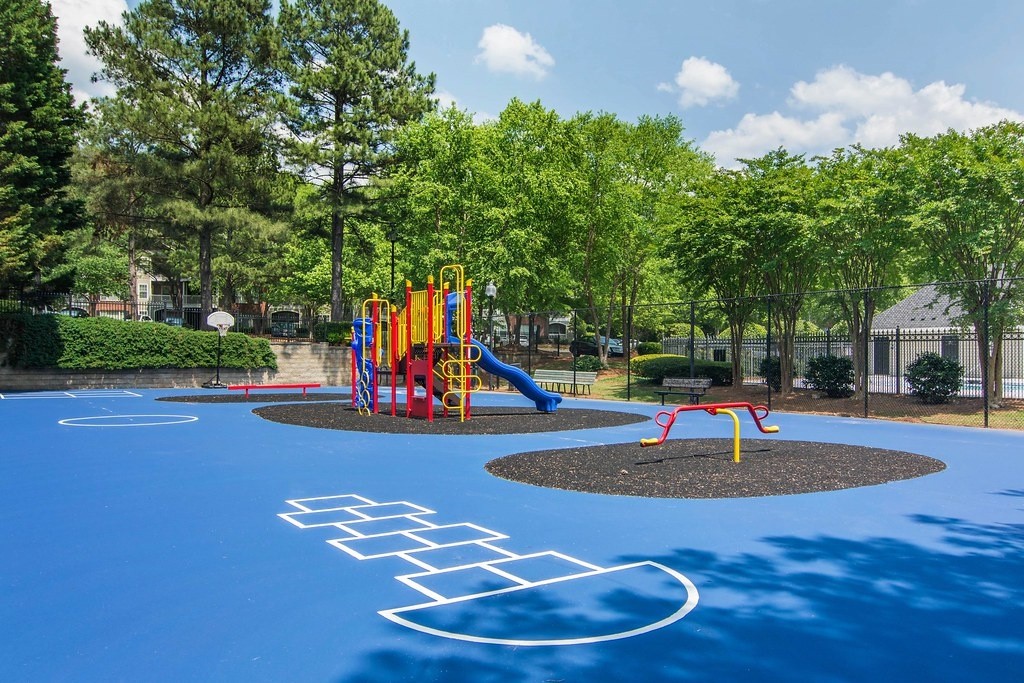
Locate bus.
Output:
[268,309,302,338]
[548,323,568,343]
[318,314,329,321]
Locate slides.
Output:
[451,336,562,413]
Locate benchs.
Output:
[228,383,321,398]
[532,369,598,395]
[654,378,712,405]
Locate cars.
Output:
[498,337,508,342]
[35,305,60,315]
[569,336,624,357]
[122,314,154,322]
[619,339,643,350]
[482,333,494,346]
[58,307,90,318]
[163,317,187,327]
[511,335,529,347]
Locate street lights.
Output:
[386,226,398,290]
[487,279,495,392]
[766,293,774,411]
